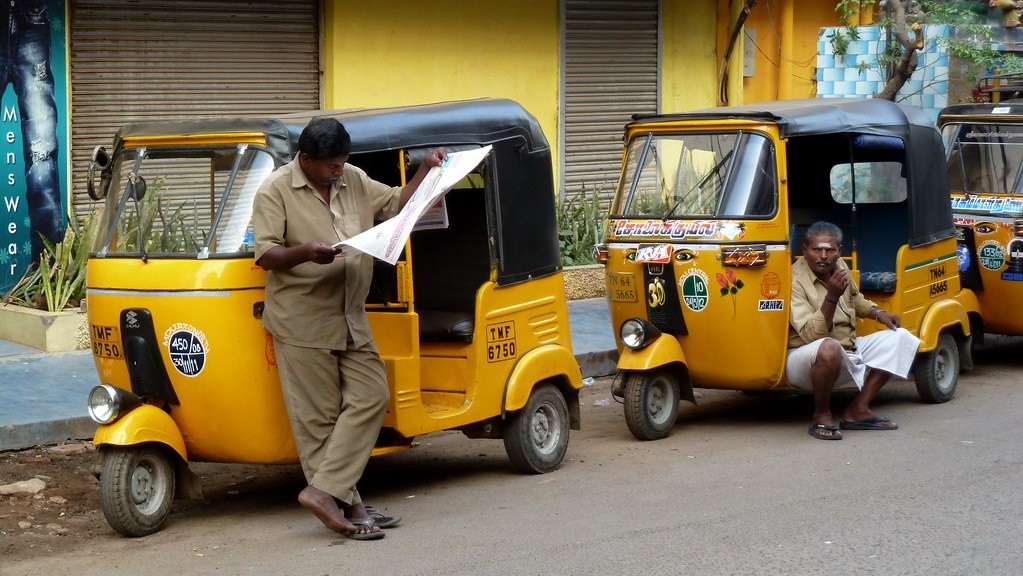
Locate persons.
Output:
[0,0,65,271]
[253,117,448,541]
[785,221,900,440]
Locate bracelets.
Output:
[875,310,887,323]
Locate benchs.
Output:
[788,202,909,296]
[364,189,482,345]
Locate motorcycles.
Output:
[932,72,1023,355]
[603,96,974,442]
[80,99,588,538]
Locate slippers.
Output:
[839,417,898,429]
[365,506,401,527]
[808,422,843,440]
[340,515,386,540]
